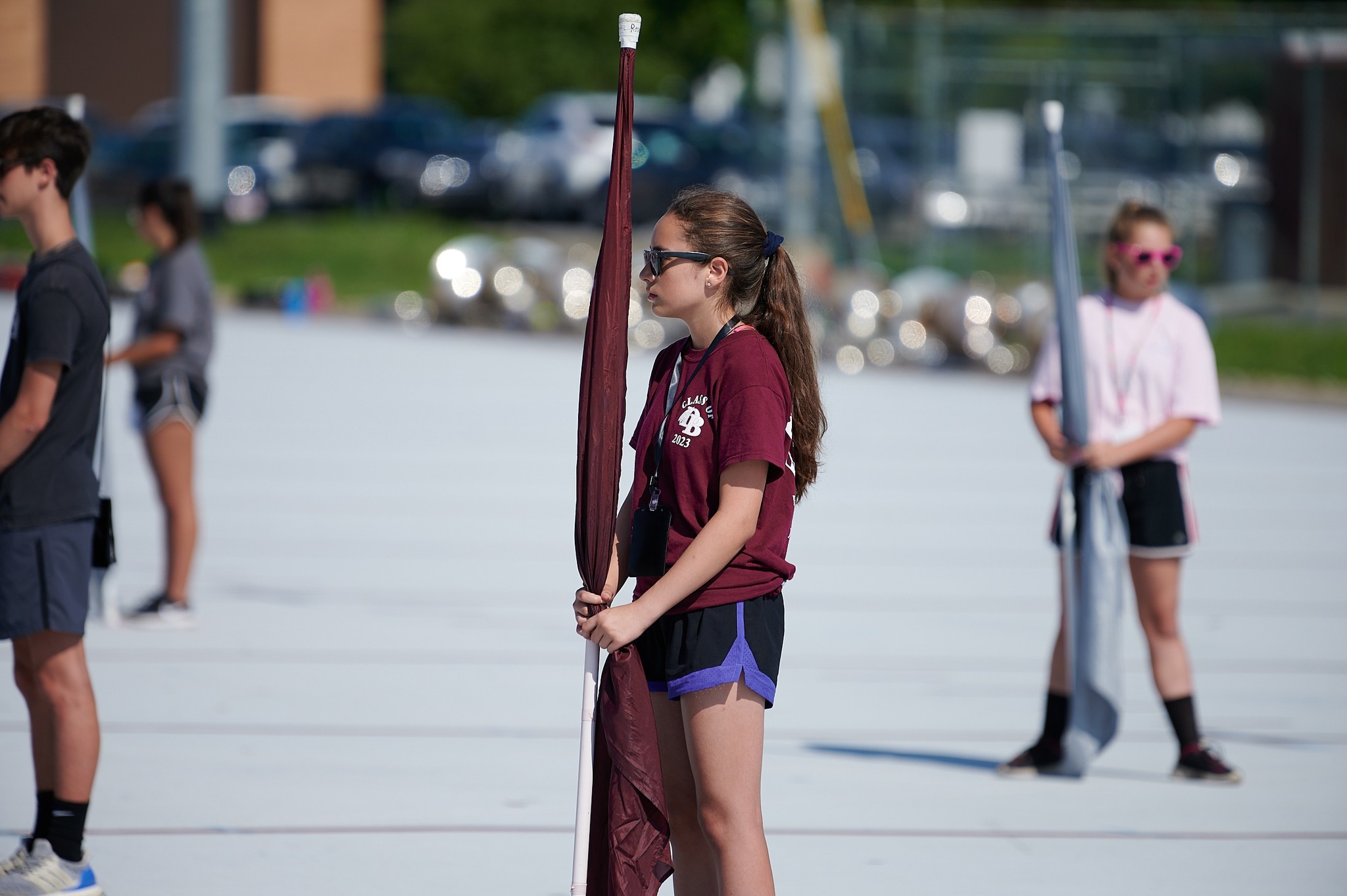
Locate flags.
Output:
[1042,102,1121,780]
[579,13,678,895]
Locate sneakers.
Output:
[0,837,100,896]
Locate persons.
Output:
[104,176,213,624]
[0,106,110,896]
[573,188,829,896]
[1003,202,1248,787]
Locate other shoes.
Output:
[996,749,1060,779]
[126,594,205,630]
[1174,745,1240,783]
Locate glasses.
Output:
[643,249,713,278]
[1117,242,1183,268]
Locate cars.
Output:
[76,82,1283,233]
